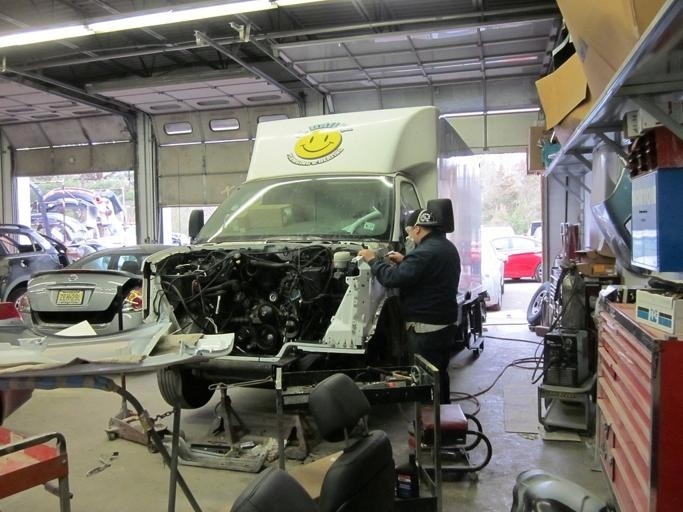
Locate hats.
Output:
[405,209,443,227]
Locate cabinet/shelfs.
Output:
[541,0,683,206]
[592,296,681,510]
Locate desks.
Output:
[5,332,233,511]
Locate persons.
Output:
[357,208,461,435]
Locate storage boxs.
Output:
[556,2,668,100]
[530,126,553,172]
[535,50,593,150]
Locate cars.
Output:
[30,188,131,249]
[489,237,543,282]
[4,224,73,311]
[24,241,188,340]
[483,229,509,313]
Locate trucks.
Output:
[156,105,491,410]
